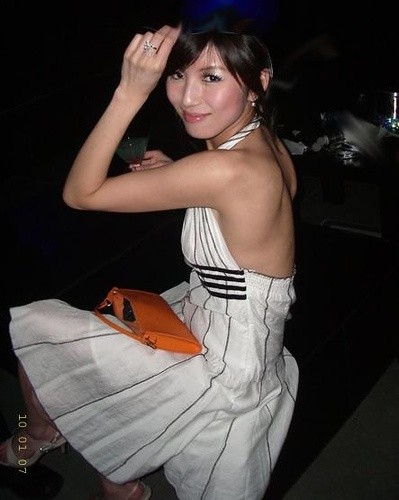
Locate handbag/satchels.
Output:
[95,286,203,354]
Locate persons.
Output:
[0,1,300,500]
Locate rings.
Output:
[143,40,158,54]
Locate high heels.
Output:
[0,429,68,470]
[126,478,152,500]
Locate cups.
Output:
[114,134,148,170]
